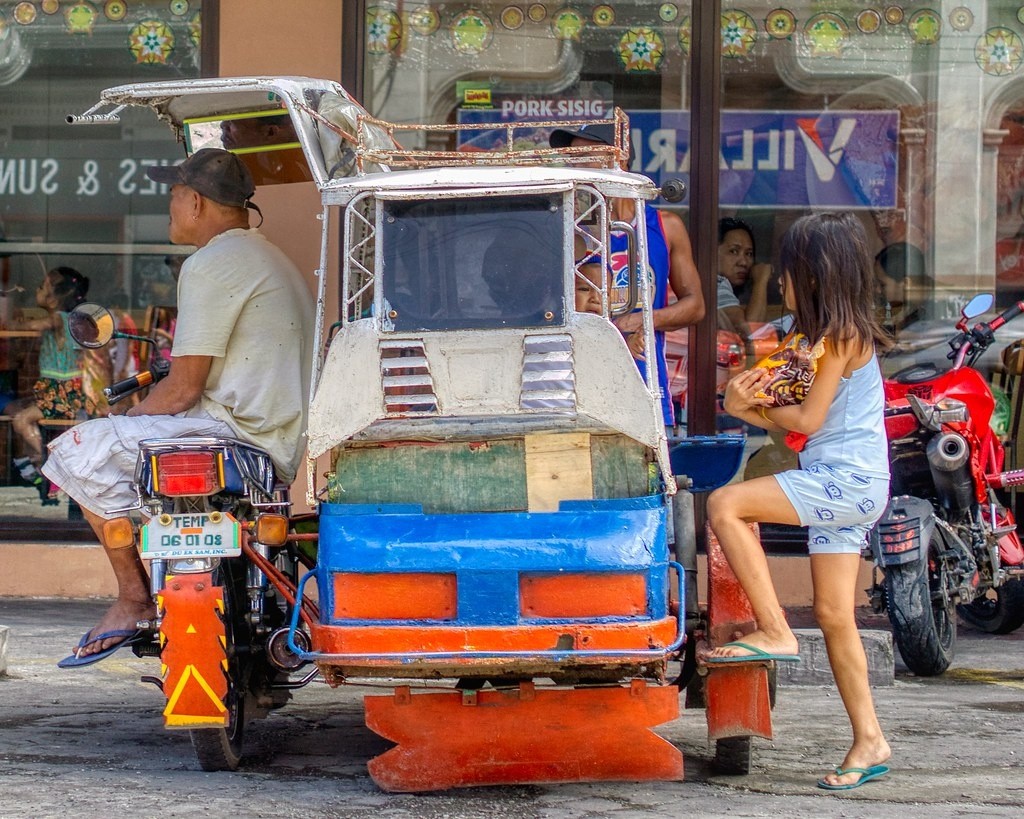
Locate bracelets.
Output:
[762,407,774,425]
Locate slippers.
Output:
[708,641,801,664]
[818,764,893,790]
[57,628,148,670]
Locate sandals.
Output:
[12,460,42,485]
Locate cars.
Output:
[879,302,1024,384]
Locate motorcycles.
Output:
[63,299,320,772]
[873,291,1024,680]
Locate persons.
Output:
[220,114,312,186]
[874,242,968,326]
[717,218,774,332]
[40,149,316,669]
[149,307,177,364]
[576,252,613,314]
[482,122,705,546]
[699,211,893,790]
[0,267,91,480]
[110,294,141,415]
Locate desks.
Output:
[0,329,42,337]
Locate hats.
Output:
[146,148,265,229]
[575,249,614,278]
[548,119,637,168]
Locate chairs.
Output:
[36,303,171,507]
[0,413,16,486]
[981,333,1024,521]
